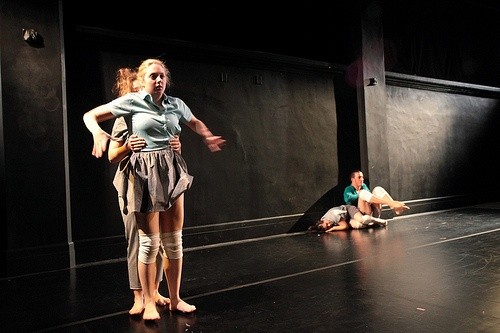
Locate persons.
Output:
[344,170,410,218]
[82,59,225,321]
[316,205,387,232]
[108,67,182,316]
